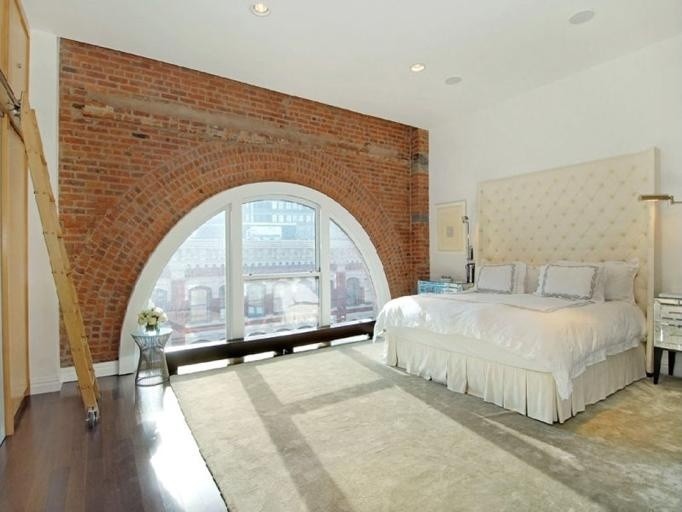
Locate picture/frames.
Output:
[435,200,468,253]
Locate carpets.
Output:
[169,339,681,512]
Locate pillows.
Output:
[473,262,528,293]
[605,256,642,303]
[534,262,605,303]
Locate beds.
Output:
[371,146,660,426]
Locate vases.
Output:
[145,326,160,335]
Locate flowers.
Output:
[137,307,166,325]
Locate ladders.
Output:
[19,90,101,430]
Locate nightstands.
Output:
[417,280,473,294]
[653,292,682,385]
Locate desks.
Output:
[129,326,174,387]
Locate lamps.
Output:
[637,194,682,206]
